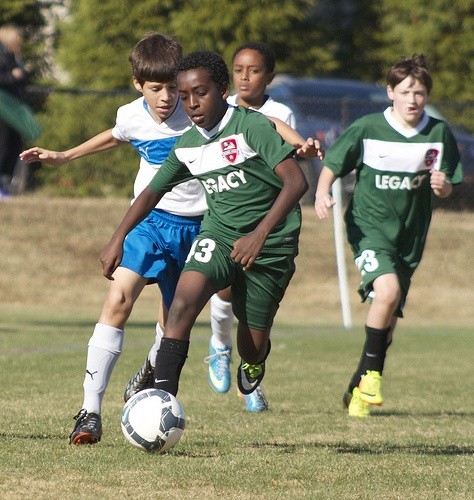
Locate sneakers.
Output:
[237,384,270,414]
[347,388,370,418]
[358,370,384,406]
[237,338,272,394]
[204,336,234,395]
[125,360,155,402]
[71,409,102,443]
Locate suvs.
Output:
[265,73,474,207]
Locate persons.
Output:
[101,51,308,396]
[18,29,323,445]
[0,15,38,200]
[314,54,463,416]
[209,43,297,412]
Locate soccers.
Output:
[121,388,185,453]
